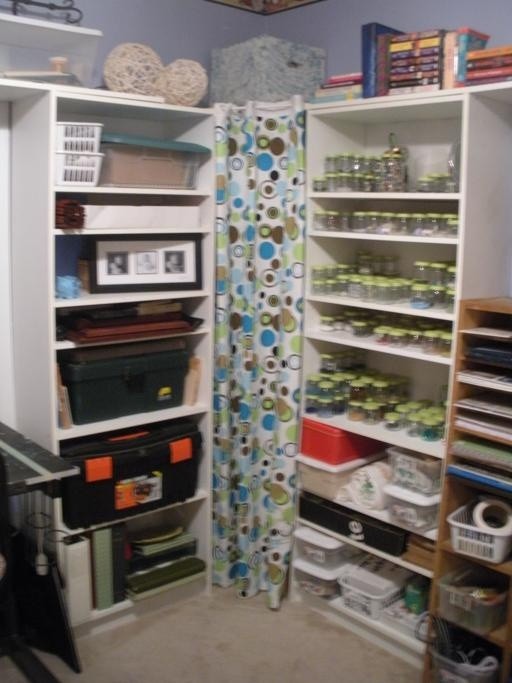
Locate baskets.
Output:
[55,121,105,185]
[447,502,511,564]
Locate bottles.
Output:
[301,134,459,442]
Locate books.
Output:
[307,22,512,107]
[445,326,512,496]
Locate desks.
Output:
[1,421,84,683]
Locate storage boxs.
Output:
[291,448,401,499]
[0,10,109,93]
[384,486,440,533]
[54,418,208,532]
[291,548,372,599]
[425,641,505,683]
[299,418,390,466]
[208,33,330,105]
[97,129,213,190]
[291,522,365,568]
[338,551,422,622]
[57,336,196,426]
[431,558,511,637]
[381,442,442,497]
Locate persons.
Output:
[166,253,183,274]
[107,255,126,275]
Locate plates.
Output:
[2,70,72,83]
[128,524,185,544]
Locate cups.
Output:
[49,56,69,83]
[56,254,89,298]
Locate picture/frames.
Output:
[82,233,204,294]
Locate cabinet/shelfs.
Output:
[420,293,512,679]
[11,86,215,647]
[289,76,511,670]
